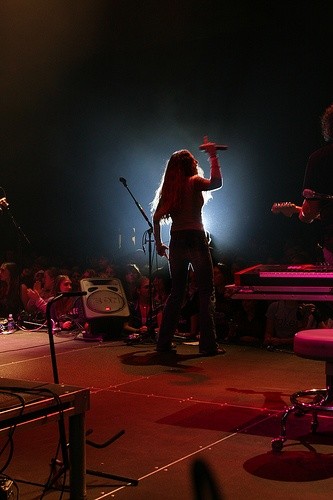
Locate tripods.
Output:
[127,183,159,345]
[41,294,138,492]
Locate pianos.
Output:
[223,263,333,347]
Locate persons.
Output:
[296,102,333,328]
[0,256,333,344]
[152,136,227,357]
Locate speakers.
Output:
[81,278,130,336]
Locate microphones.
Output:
[56,292,87,296]
[199,144,228,150]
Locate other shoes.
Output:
[157,347,177,355]
[204,347,225,355]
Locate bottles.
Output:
[8,314,14,332]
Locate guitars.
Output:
[271,202,302,217]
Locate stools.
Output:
[271,329,333,451]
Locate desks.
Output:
[0,378,90,500]
